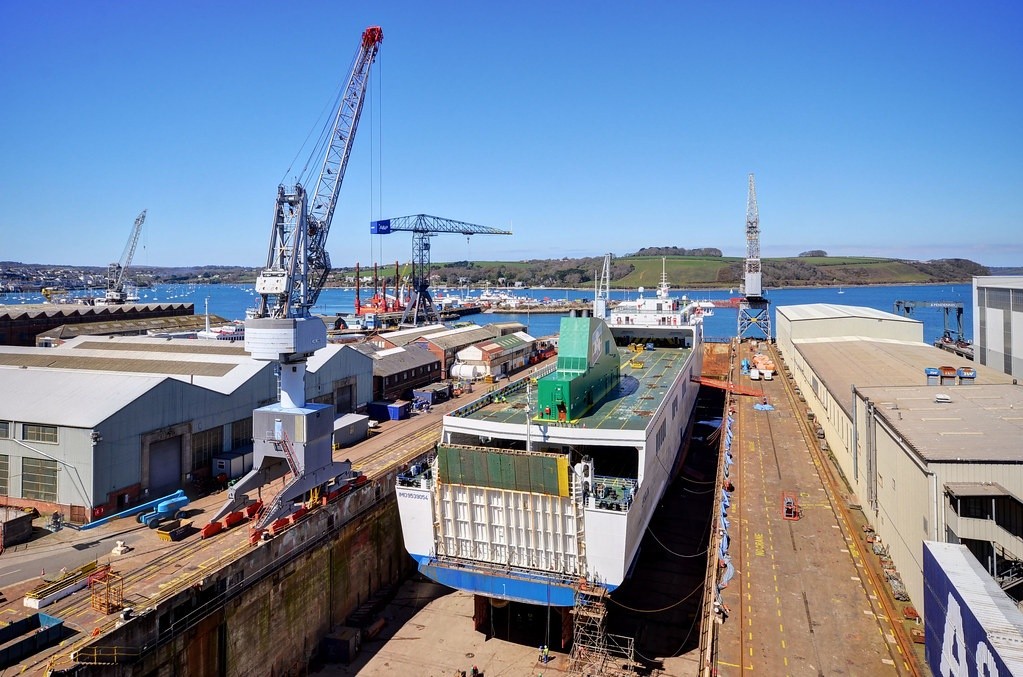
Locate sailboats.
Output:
[388,284,582,312]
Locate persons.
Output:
[538,645,550,663]
[53,510,60,527]
[469,666,478,677]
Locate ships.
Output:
[394,253,722,609]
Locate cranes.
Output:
[204,20,419,546]
[105,210,149,303]
[370,214,513,324]
[737,172,772,342]
